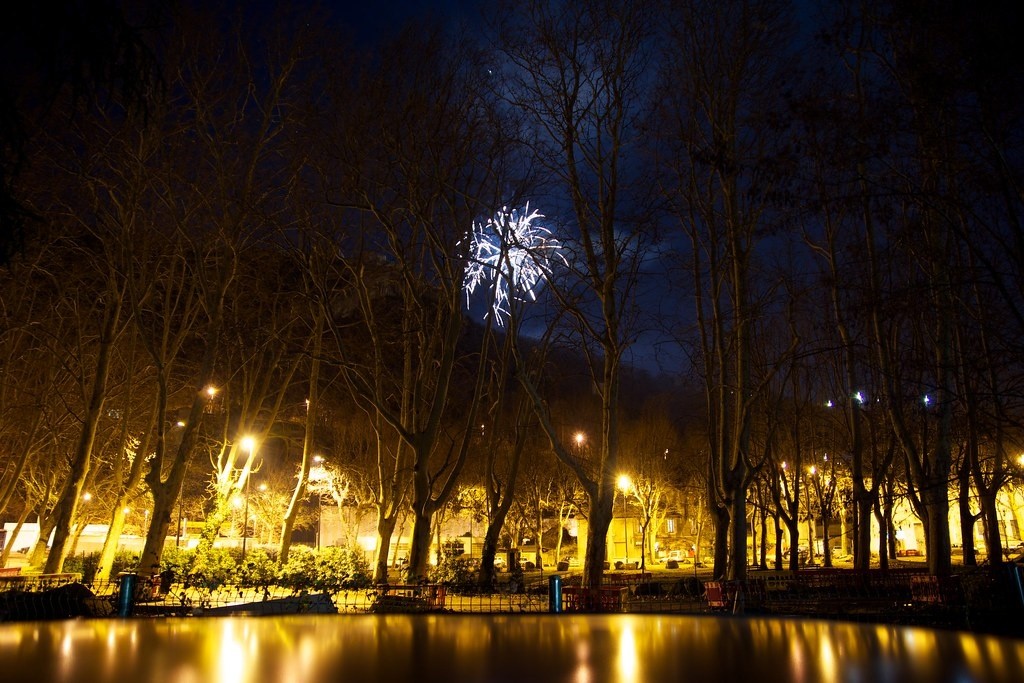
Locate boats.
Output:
[367,579,446,616]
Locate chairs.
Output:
[704,581,733,611]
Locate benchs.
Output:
[377,583,447,607]
[562,586,631,612]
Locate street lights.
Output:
[314,453,326,553]
[239,435,257,551]
[770,451,1024,558]
[122,509,131,533]
[141,511,151,535]
[83,493,94,538]
[619,477,634,568]
